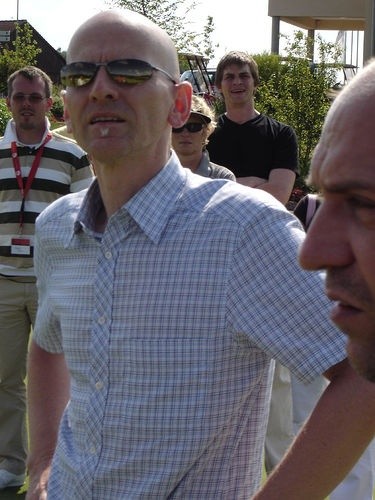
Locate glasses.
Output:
[60,59,178,87]
[172,122,205,133]
[10,92,47,104]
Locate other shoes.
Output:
[0,469,25,488]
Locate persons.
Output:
[24,7,375,500]
[0,66,94,489]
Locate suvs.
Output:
[179,67,224,104]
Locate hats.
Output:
[189,109,212,124]
